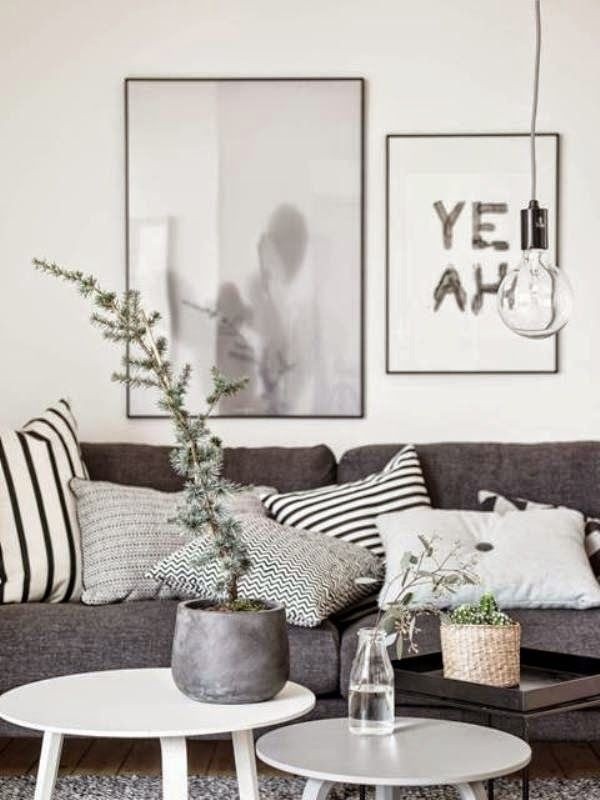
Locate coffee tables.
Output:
[256,716,532,800]
[0,668,316,800]
[394,638,600,800]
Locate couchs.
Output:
[0,442,600,743]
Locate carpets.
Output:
[1,774,600,800]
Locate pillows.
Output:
[0,396,600,628]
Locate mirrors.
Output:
[124,77,364,420]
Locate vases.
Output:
[348,628,395,734]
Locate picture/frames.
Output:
[385,134,560,375]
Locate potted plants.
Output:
[32,257,290,706]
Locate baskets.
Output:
[440,620,522,689]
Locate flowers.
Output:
[353,531,494,661]
